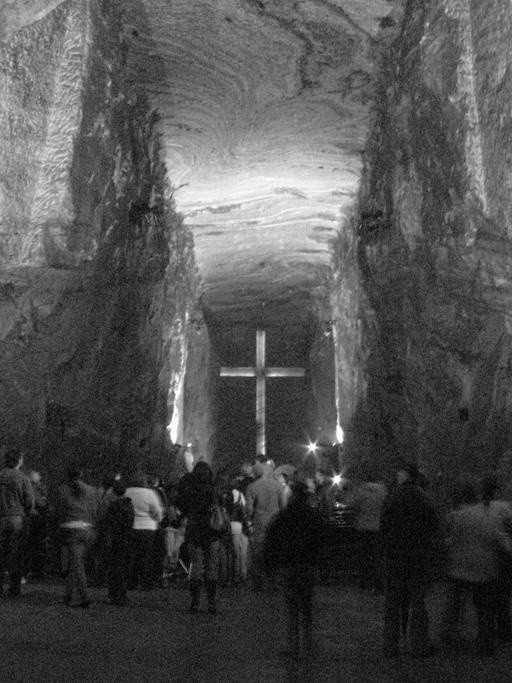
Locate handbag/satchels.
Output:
[210,504,232,535]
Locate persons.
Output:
[0,446,512,662]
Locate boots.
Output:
[190,578,218,615]
